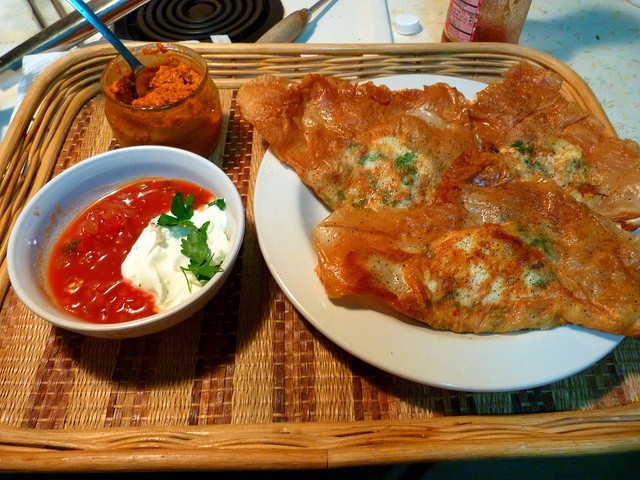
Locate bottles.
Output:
[440,0,531,46]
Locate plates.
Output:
[253,74,639,394]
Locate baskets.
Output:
[0,42,639,471]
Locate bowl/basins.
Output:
[7,145,245,340]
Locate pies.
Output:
[231,56,640,344]
